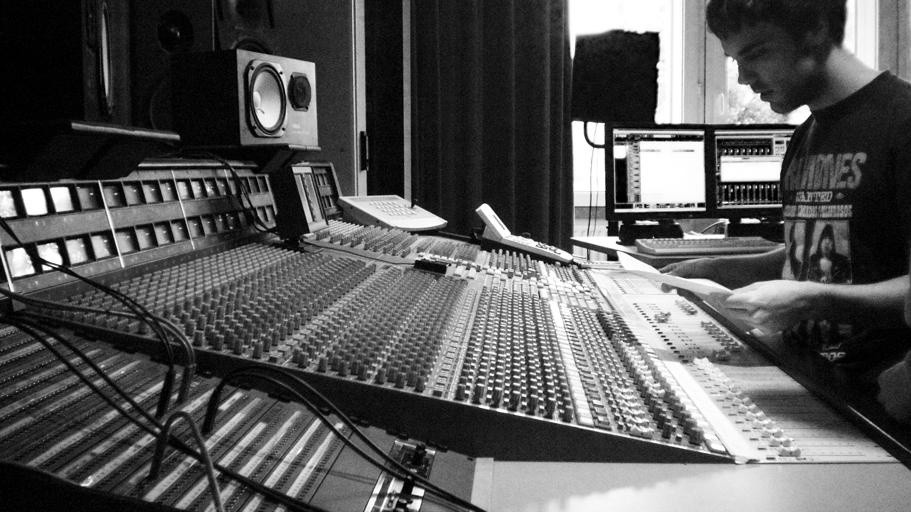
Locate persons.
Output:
[807,220,853,339]
[878,349,910,425]
[659,0,910,445]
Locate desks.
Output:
[569,233,789,269]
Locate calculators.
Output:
[476,204,573,264]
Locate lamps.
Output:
[566,27,662,150]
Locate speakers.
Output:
[0,0,321,183]
[571,29,659,130]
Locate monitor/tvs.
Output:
[712,124,800,242]
[605,120,713,246]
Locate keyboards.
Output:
[634,235,783,255]
